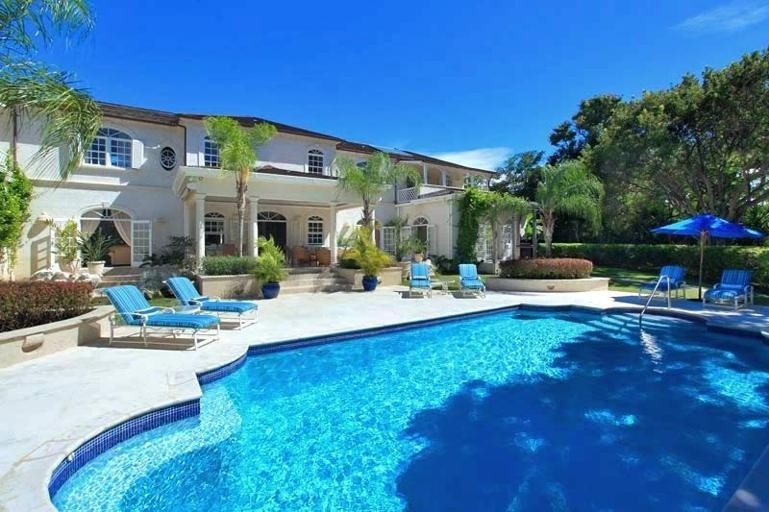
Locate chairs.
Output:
[640,264,754,311]
[100,277,258,352]
[409,263,487,299]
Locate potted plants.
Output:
[341,227,394,291]
[247,235,289,299]
[37,218,122,276]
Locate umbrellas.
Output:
[649,211,764,300]
